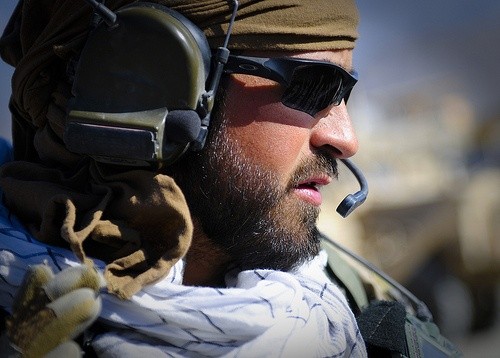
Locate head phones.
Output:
[66,0,239,169]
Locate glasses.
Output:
[227,54,358,117]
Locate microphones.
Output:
[336,158,370,217]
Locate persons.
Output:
[0,1,461,357]
